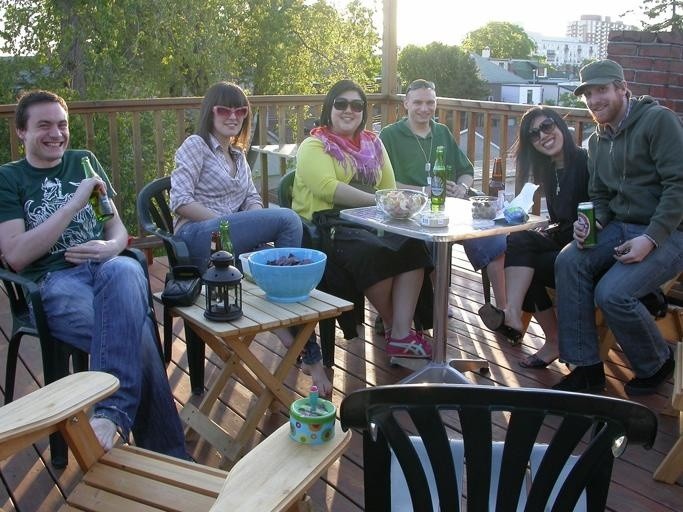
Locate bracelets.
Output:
[421,186,425,193]
[461,183,469,194]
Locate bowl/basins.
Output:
[246,247,328,303]
[470,195,499,228]
[374,187,428,219]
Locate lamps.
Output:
[201,251,242,322]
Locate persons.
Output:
[0,90,189,460]
[170,80,332,398]
[477,105,591,368]
[550,59,683,395]
[290,80,433,358]
[374,79,507,336]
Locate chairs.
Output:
[0,368,353,512]
[339,383,657,512]
[136,175,304,396]
[276,169,422,370]
[0,247,163,470]
[444,241,491,305]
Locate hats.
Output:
[573,57,625,95]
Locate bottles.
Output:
[81,155,116,222]
[488,156,505,207]
[445,164,454,183]
[208,216,236,270]
[431,144,448,205]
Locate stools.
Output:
[655,340,683,485]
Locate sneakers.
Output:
[624,346,676,397]
[384,328,434,359]
[88,414,117,452]
[553,362,607,394]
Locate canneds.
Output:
[576,202,598,248]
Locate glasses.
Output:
[213,105,249,119]
[525,119,558,144]
[334,98,364,112]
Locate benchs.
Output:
[518,277,683,363]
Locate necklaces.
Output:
[555,166,560,196]
[414,132,433,184]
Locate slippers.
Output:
[519,348,564,369]
[478,302,505,330]
[499,323,523,346]
